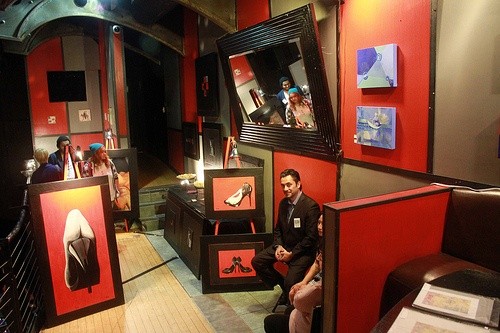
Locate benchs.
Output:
[376,182,500,320]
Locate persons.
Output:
[264,212,323,333]
[277,76,317,129]
[30,135,119,209]
[251,169,320,315]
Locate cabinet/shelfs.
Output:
[166,188,202,280]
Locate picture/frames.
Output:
[84,147,139,221]
[183,120,199,160]
[201,233,275,294]
[31,175,126,327]
[202,122,224,167]
[196,52,220,117]
[204,167,265,218]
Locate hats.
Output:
[89,143,102,154]
[288,87,299,95]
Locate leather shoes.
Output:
[277,293,289,305]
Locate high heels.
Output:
[222,256,253,274]
[224,182,253,208]
[62,208,101,293]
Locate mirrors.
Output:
[230,41,319,127]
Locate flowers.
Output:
[194,182,204,190]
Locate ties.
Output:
[287,205,296,223]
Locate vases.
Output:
[196,187,205,201]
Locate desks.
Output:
[374,269,500,333]
